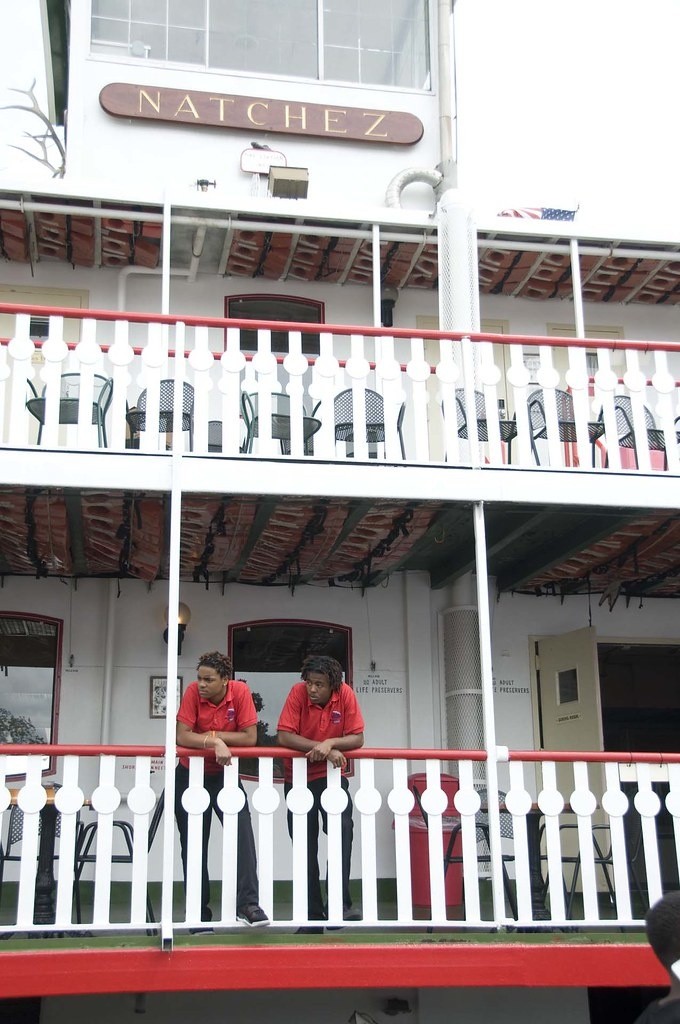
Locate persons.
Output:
[635,892,680,1024]
[176,651,270,935]
[276,656,364,934]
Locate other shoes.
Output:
[294,911,326,935]
[326,909,361,930]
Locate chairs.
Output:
[26,371,680,476]
[0,782,168,943]
[412,783,661,934]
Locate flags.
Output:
[497,208,575,221]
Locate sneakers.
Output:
[236,905,269,928]
[189,913,216,936]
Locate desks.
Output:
[7,788,93,927]
[482,801,601,936]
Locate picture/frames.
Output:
[149,676,183,719]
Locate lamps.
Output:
[163,601,192,656]
[268,165,309,201]
[348,999,411,1024]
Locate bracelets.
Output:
[213,731,216,737]
[204,736,208,748]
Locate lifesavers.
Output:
[386,166,442,224]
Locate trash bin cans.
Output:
[390,773,463,907]
